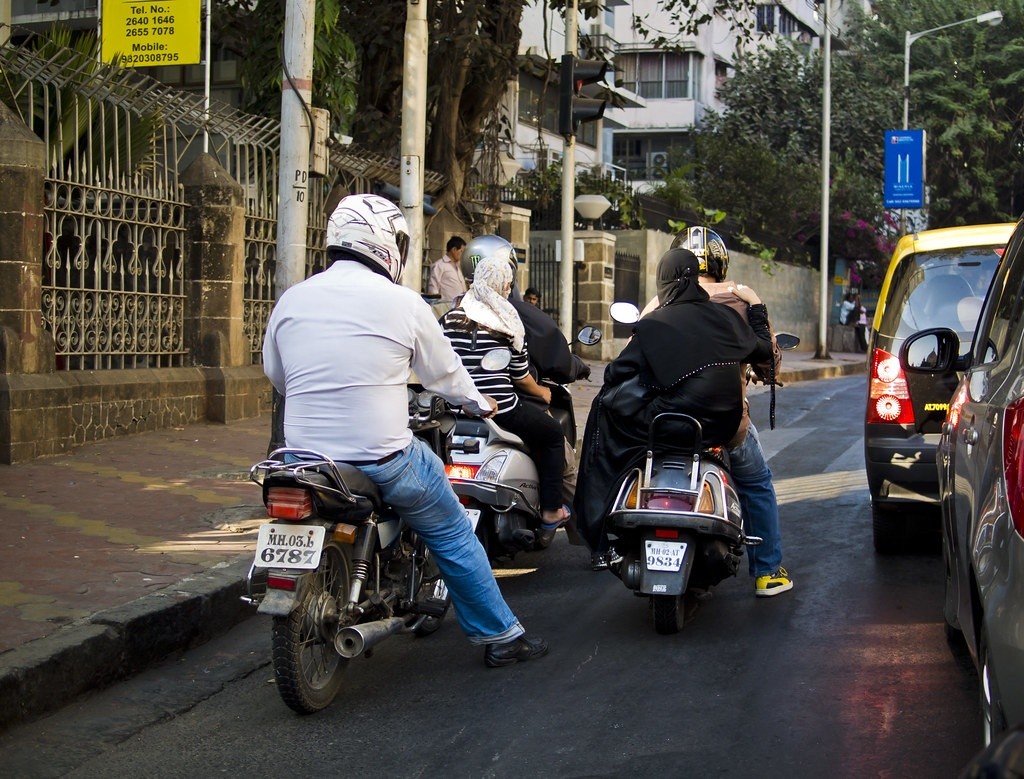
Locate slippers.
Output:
[541,504,571,531]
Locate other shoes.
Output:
[590,547,623,571]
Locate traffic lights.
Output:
[559,54,607,135]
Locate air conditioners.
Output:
[646,168,668,180]
[646,151,668,168]
[591,24,611,54]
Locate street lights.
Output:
[899,10,1004,239]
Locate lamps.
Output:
[475,151,522,200]
[574,194,613,229]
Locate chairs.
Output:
[921,276,975,331]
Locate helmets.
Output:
[669,226,729,283]
[460,235,518,287]
[325,193,410,284]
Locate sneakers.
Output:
[755,567,794,596]
[484,636,550,668]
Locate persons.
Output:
[261,194,550,667]
[456,233,590,512]
[522,287,541,307]
[589,330,600,343]
[639,225,794,599]
[572,248,775,573]
[438,258,573,532]
[431,235,467,302]
[839,292,866,350]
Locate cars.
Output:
[856,212,1024,779]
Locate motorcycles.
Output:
[239,325,602,714]
[609,302,801,634]
